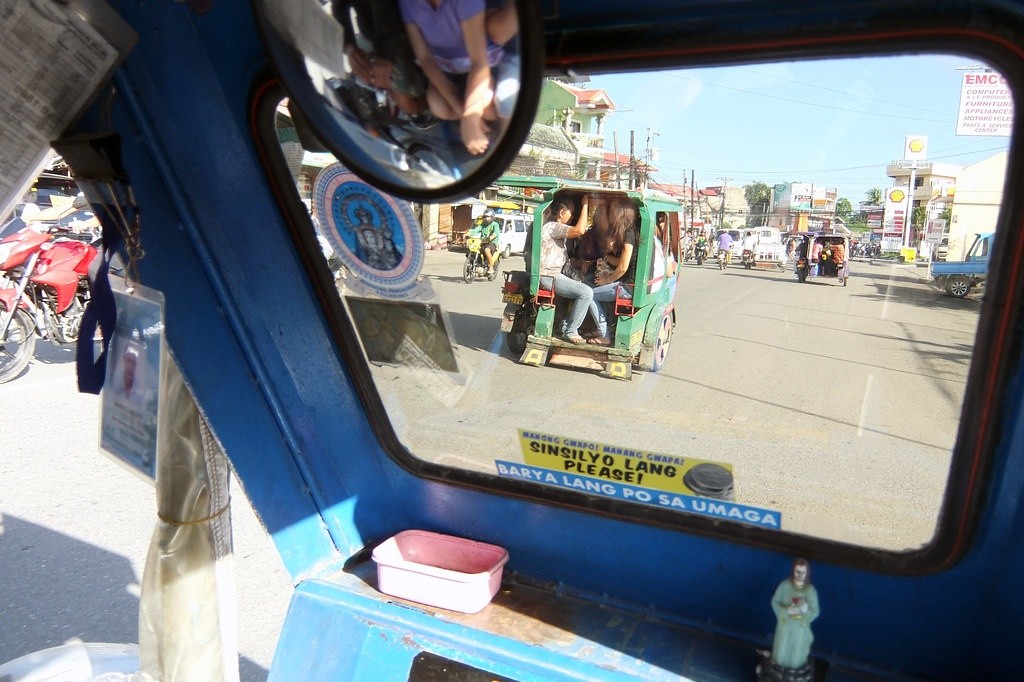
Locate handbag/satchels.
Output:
[594,258,616,279]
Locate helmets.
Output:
[700,229,706,233]
[482,208,495,225]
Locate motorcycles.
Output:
[500,270,538,356]
[795,257,811,284]
[462,232,503,284]
[693,240,708,266]
[495,173,684,381]
[0,211,107,385]
[741,246,756,269]
[716,244,733,271]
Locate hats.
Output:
[724,228,729,233]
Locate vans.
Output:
[492,212,535,260]
[710,226,872,278]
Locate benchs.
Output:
[811,263,821,275]
[837,264,843,276]
[615,276,663,318]
[536,275,571,307]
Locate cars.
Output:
[4,168,106,245]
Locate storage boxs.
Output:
[371,530,512,615]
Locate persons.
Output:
[695,229,708,259]
[465,209,499,273]
[741,232,755,263]
[814,237,844,276]
[540,193,674,346]
[716,230,734,264]
[22,202,103,283]
[788,239,795,261]
[687,235,696,255]
[330,0,518,156]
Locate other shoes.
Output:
[728,262,732,265]
[487,270,495,274]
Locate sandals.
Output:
[562,335,586,345]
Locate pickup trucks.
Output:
[930,231,995,298]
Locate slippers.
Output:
[587,338,611,346]
